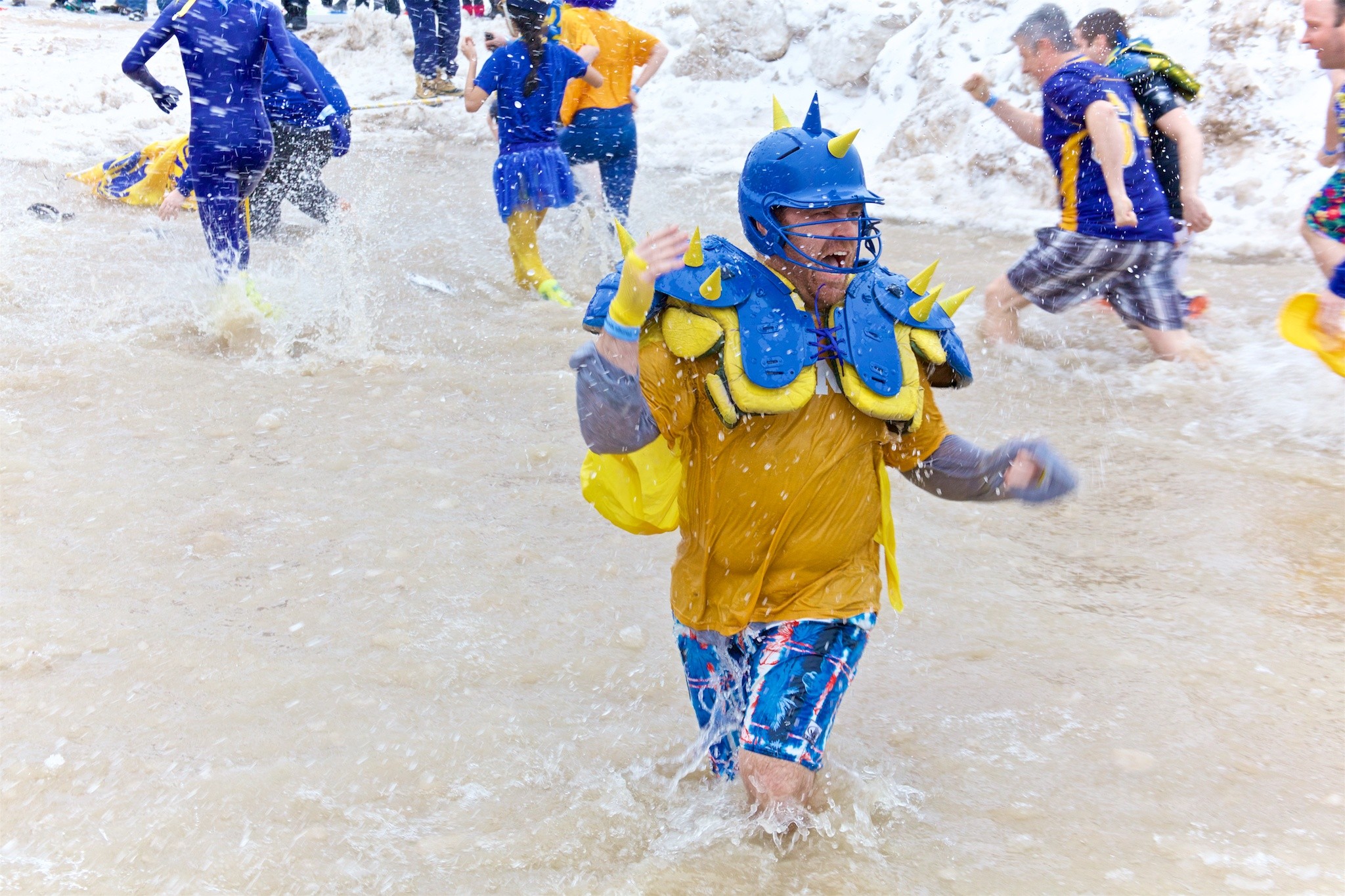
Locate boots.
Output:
[415,73,443,107]
[435,67,465,97]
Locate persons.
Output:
[152,28,354,247]
[122,0,347,282]
[1282,64,1345,382]
[460,1,604,308]
[0,0,506,25]
[568,90,1077,850]
[1298,0,1344,73]
[1072,8,1213,331]
[402,0,467,107]
[545,0,669,240]
[961,3,1213,370]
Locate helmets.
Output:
[737,90,886,274]
[506,0,553,15]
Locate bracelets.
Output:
[605,314,641,340]
[631,85,641,93]
[1322,145,1338,155]
[984,95,999,107]
